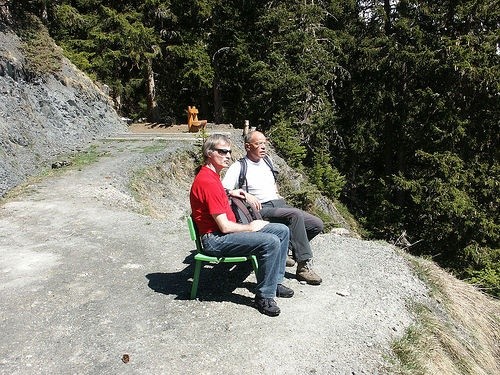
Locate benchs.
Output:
[187,106,207,133]
[188,197,261,300]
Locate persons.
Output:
[189,134,294,316]
[222,131,324,284]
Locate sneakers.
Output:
[276,283,294,298]
[286,255,295,267]
[254,295,280,316]
[296,261,322,284]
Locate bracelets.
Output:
[226,189,231,194]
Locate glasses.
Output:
[214,149,232,154]
